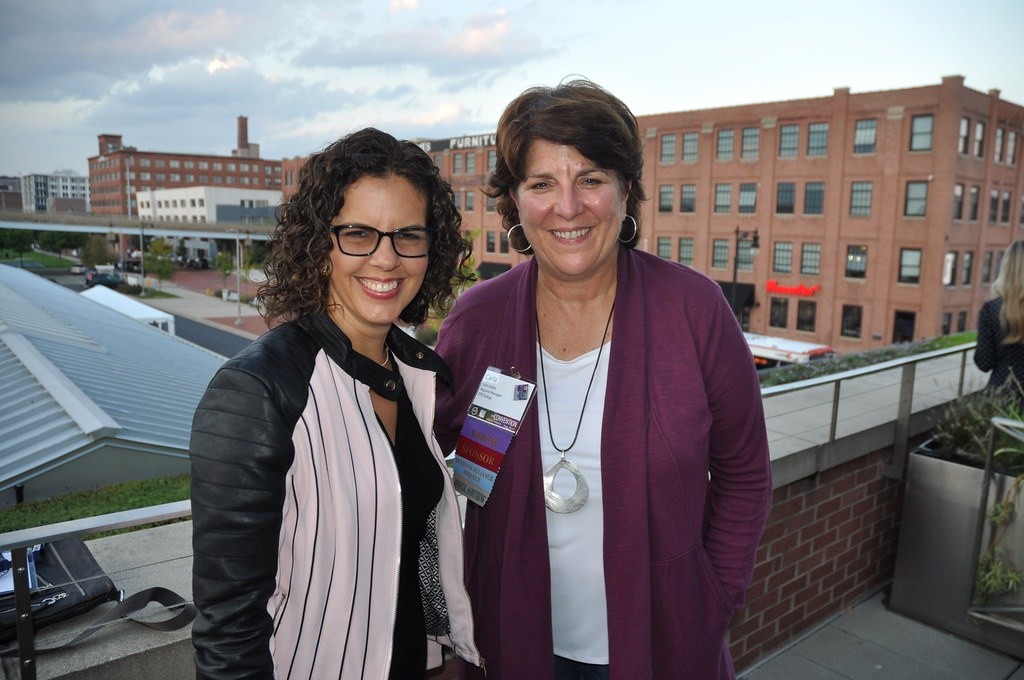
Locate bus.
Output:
[741,331,835,373]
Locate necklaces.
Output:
[382,343,389,369]
[535,295,618,514]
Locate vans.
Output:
[124,258,142,273]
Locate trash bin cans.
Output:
[222,288,229,301]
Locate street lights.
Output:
[224,226,246,325]
[138,220,153,296]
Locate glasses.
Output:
[329,224,439,258]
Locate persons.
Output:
[433,75,776,680]
[973,241,1024,399]
[190,128,487,680]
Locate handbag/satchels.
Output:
[0,537,118,641]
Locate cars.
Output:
[70,264,89,274]
[85,271,126,291]
[189,258,209,269]
